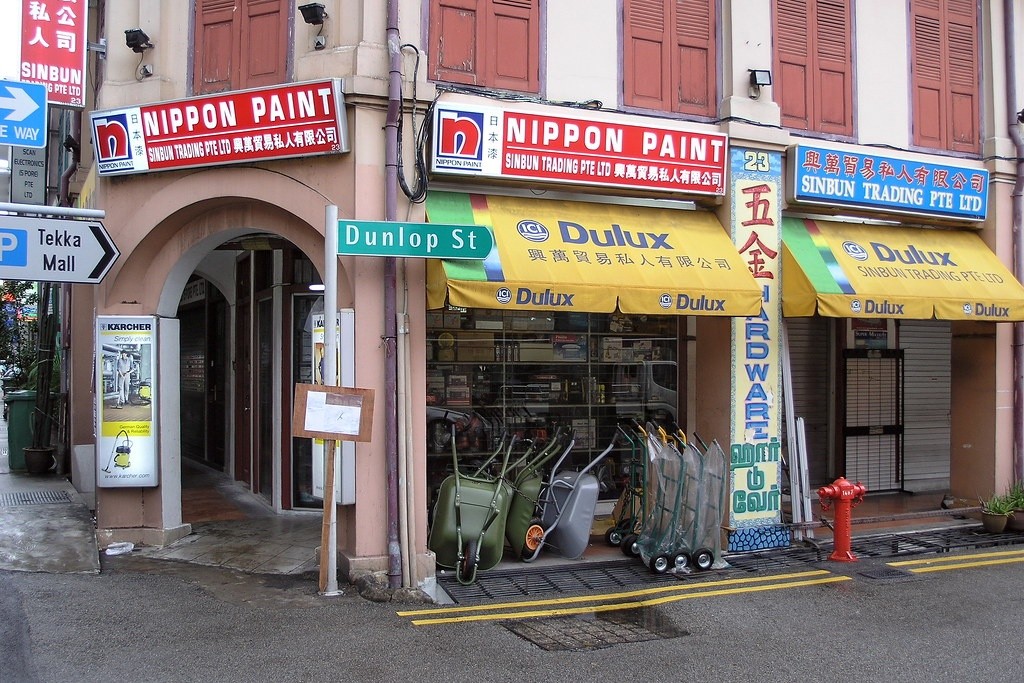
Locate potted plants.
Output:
[1005,478,1024,532]
[976,491,1016,533]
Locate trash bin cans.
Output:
[2,390,59,473]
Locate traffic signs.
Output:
[1,79,49,150]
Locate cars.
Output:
[426,406,473,453]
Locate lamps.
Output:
[747,68,772,84]
[124,28,154,53]
[297,2,329,25]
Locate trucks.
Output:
[496,360,678,445]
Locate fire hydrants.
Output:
[818,476,868,562]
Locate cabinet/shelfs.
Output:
[426,306,679,510]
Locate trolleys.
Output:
[428,418,728,585]
